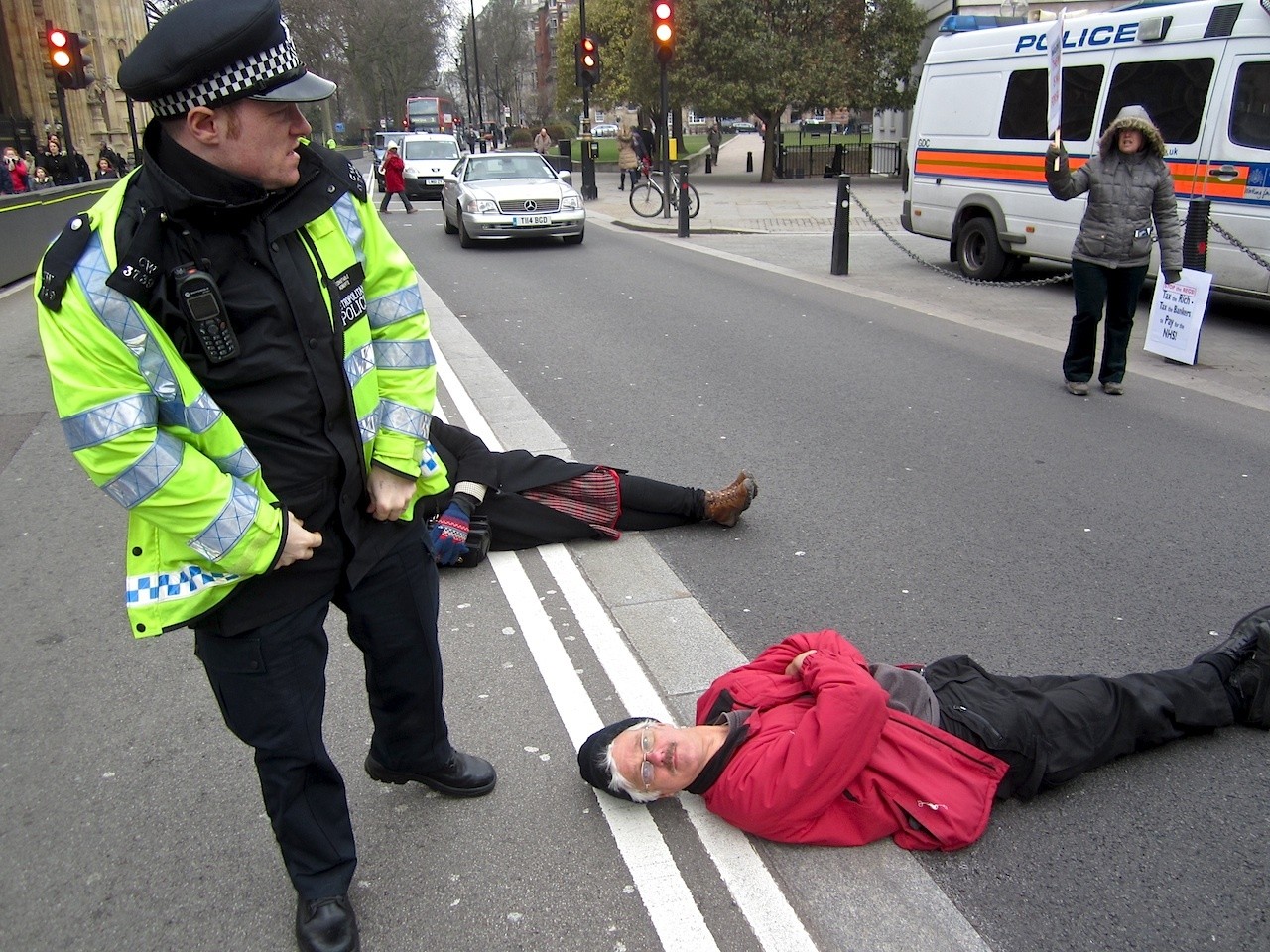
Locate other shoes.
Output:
[379,209,392,216]
[406,207,418,214]
[703,468,760,529]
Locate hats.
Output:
[576,717,661,803]
[116,1,347,121]
[386,139,397,153]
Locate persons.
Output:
[708,124,722,166]
[34,0,496,952]
[534,127,551,154]
[1045,106,1183,395]
[465,127,477,153]
[423,415,759,568]
[618,124,656,191]
[380,139,418,215]
[0,133,127,196]
[327,137,336,150]
[489,123,495,134]
[578,606,1270,853]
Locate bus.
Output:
[477,120,495,141]
[403,96,457,136]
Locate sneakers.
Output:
[1100,380,1125,395]
[1063,378,1089,396]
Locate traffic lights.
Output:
[46,28,95,91]
[575,36,600,88]
[650,0,676,63]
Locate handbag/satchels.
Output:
[423,510,493,569]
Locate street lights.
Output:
[459,25,475,154]
[492,50,502,144]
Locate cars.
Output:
[439,151,586,248]
[591,124,620,138]
[368,131,469,202]
[729,122,757,133]
[800,118,819,131]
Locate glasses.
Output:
[639,724,659,795]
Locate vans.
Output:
[898,0,1269,312]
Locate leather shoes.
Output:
[359,747,501,797]
[1195,605,1270,729]
[291,889,361,951]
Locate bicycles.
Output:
[628,158,701,220]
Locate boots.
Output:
[630,172,636,192]
[618,172,627,191]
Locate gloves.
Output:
[425,496,472,566]
